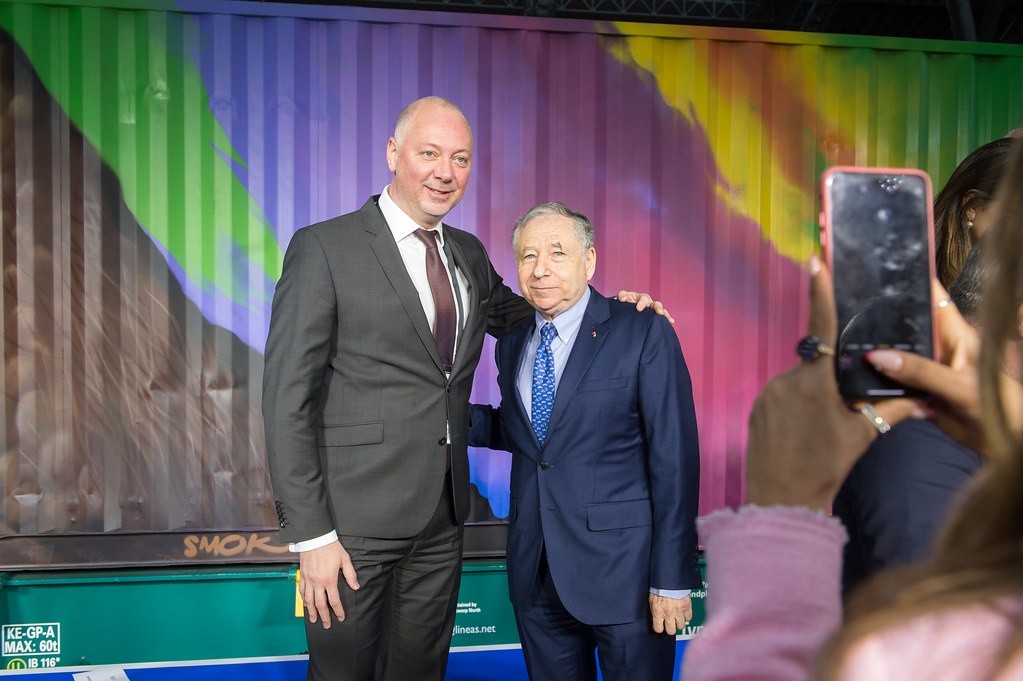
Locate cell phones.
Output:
[820,166,940,409]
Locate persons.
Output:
[684,139,1023,681]
[468,201,700,681]
[262,97,674,681]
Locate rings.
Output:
[937,298,951,308]
[862,406,890,433]
[796,335,836,361]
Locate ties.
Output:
[413,229,456,374]
[531,323,558,448]
